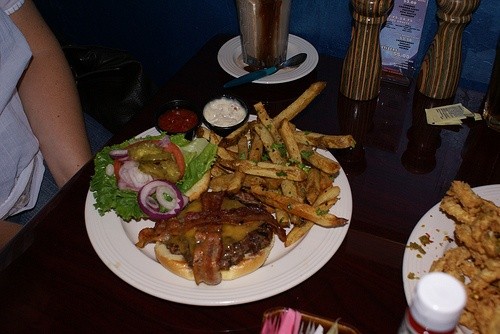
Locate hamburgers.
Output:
[89,133,287,285]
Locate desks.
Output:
[0,33,500,334]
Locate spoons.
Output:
[223,53,307,88]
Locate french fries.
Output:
[200,79,355,246]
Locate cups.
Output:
[235,0,291,69]
[478,30,500,130]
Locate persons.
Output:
[0,0,112,242]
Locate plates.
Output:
[85,114,353,308]
[217,32,320,85]
[402,184,500,334]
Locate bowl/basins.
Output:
[202,95,250,138]
[152,100,201,138]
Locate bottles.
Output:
[396,270,469,334]
